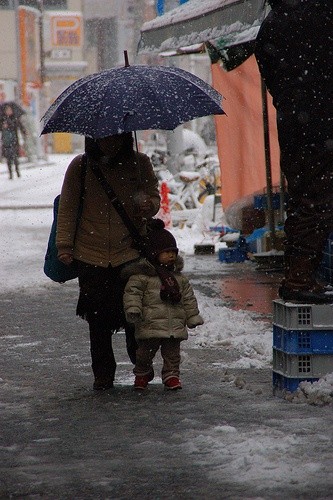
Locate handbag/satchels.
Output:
[43,152,87,284]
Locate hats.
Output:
[143,219,178,260]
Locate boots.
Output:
[278,247,333,304]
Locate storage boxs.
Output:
[270,299,333,398]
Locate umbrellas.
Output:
[0,102,25,117]
[40,50,228,194]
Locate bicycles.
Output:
[149,145,222,228]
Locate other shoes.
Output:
[164,377,182,390]
[93,379,114,391]
[147,366,154,382]
[133,375,148,389]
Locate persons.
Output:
[0,105,27,180]
[123,228,204,392]
[56,131,162,390]
